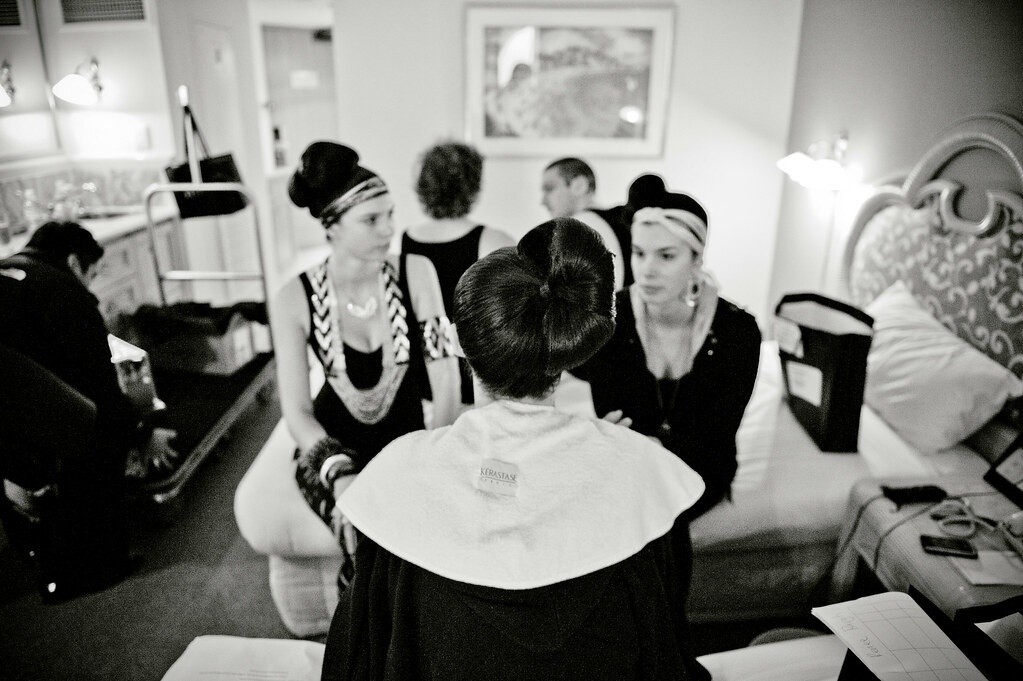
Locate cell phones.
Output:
[921,534,978,559]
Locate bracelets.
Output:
[309,435,357,489]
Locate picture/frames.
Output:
[463,5,675,159]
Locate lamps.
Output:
[0,60,15,108]
[53,60,102,106]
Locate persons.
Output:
[268,142,466,591]
[386,140,519,405]
[327,221,711,681]
[539,156,635,290]
[0,220,181,591]
[590,195,763,634]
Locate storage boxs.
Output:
[105,314,257,478]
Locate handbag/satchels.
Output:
[775,293,875,455]
[105,332,168,420]
[166,107,248,220]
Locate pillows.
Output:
[863,279,1023,457]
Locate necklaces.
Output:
[641,299,700,434]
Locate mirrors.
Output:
[0,0,65,172]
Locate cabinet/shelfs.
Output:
[86,218,191,324]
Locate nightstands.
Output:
[826,473,1023,624]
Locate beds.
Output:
[232,109,1023,639]
[157,594,1023,681]
